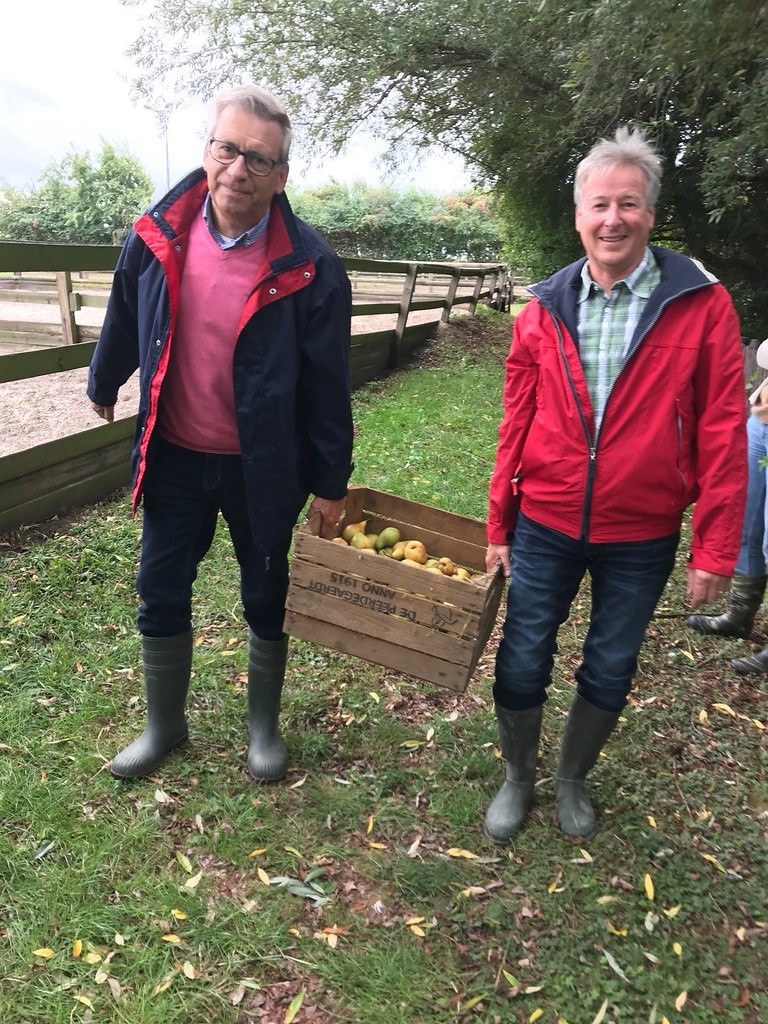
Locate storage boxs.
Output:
[281,489,506,692]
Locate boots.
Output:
[554,692,623,843]
[688,572,768,639]
[482,700,544,846]
[109,621,192,778]
[730,646,768,674]
[247,628,289,782]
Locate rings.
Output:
[717,591,723,594]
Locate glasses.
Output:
[208,136,285,176]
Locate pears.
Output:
[326,521,485,607]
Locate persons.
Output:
[483,124,743,847]
[686,334,768,676]
[86,82,355,784]
